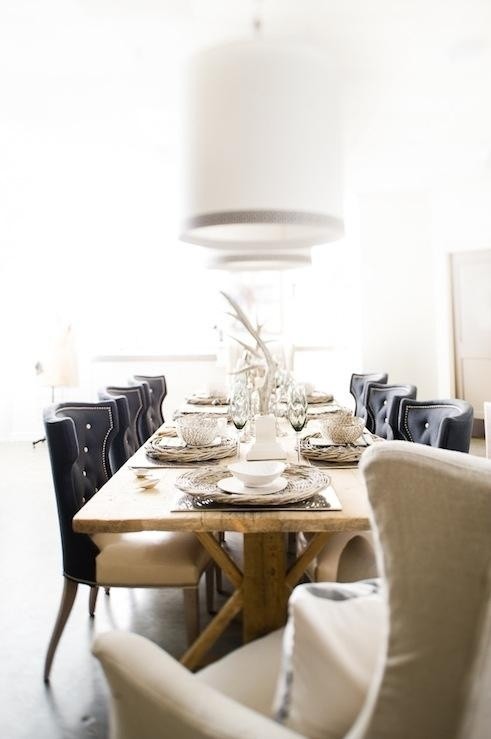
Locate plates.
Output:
[218,476,288,496]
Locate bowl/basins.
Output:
[228,462,285,489]
[173,418,220,446]
[318,415,365,444]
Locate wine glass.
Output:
[229,383,307,462]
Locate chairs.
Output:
[350,372,473,454]
[43,375,167,687]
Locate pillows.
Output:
[270,577,389,739]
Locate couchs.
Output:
[89,441,491,739]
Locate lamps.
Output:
[180,6,348,273]
